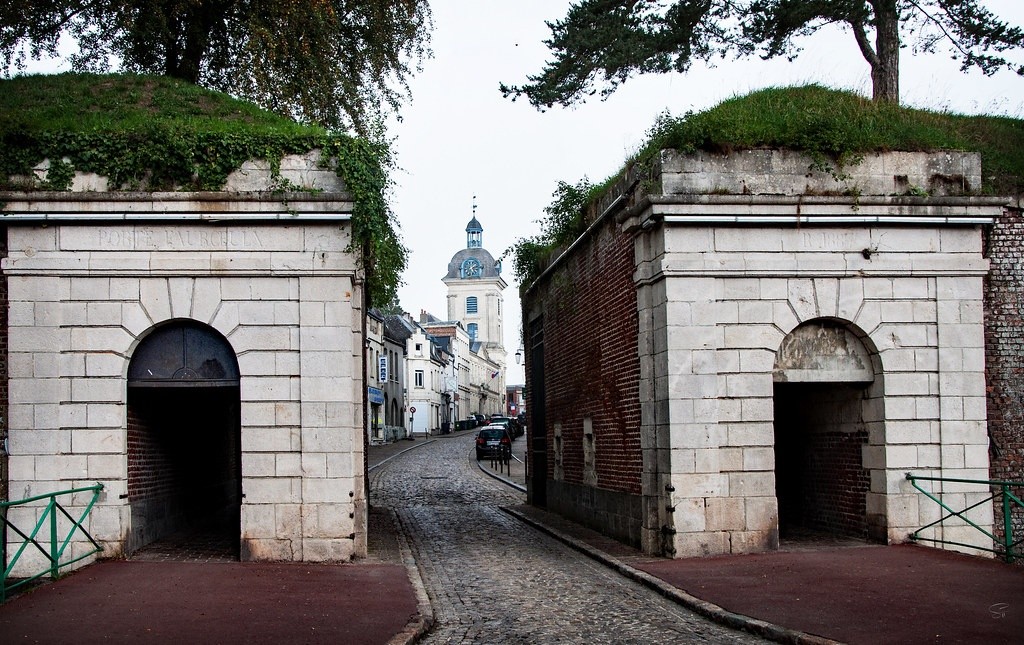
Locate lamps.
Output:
[515,349,524,364]
[463,261,478,276]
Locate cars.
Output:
[474,425,513,461]
[476,413,527,441]
